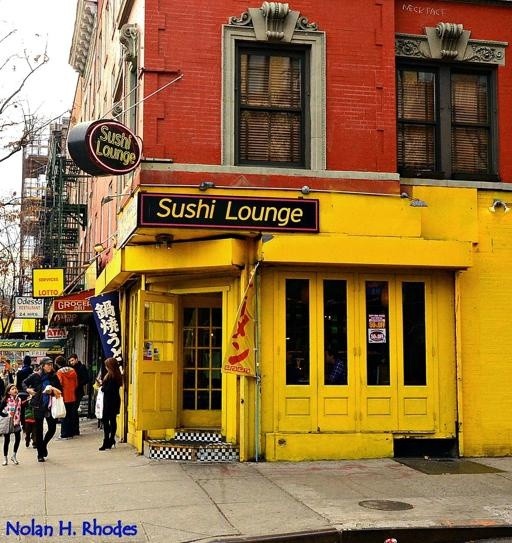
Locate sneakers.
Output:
[58,433,68,440]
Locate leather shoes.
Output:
[33,444,36,448]
[26,441,29,447]
[42,448,47,457]
[37,457,44,462]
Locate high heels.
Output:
[99,438,111,450]
[109,437,116,448]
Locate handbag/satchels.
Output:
[0,415,14,436]
[51,395,67,420]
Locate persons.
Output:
[0,354,122,466]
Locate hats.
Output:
[39,356,54,365]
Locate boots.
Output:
[11,451,18,464]
[2,456,7,465]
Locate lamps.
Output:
[401,192,428,208]
[94,229,118,247]
[100,188,135,207]
[489,199,511,213]
